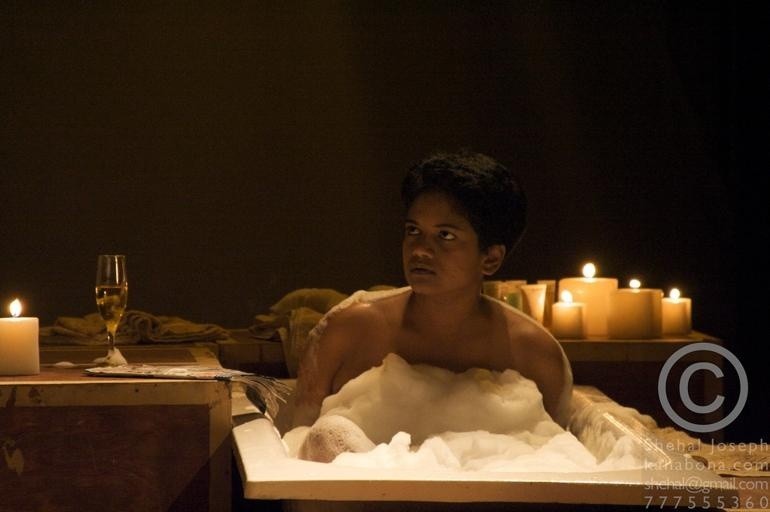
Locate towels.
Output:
[653,422,770,478]
[244,279,400,380]
[35,308,235,348]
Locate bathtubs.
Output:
[227,374,743,512]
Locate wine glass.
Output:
[93,252,130,372]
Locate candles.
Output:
[556,263,620,338]
[604,276,665,341]
[0,295,42,377]
[550,290,588,341]
[661,287,693,339]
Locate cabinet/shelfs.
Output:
[0,334,238,510]
[545,325,726,448]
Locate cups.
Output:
[485,277,557,327]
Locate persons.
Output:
[276,152,575,468]
